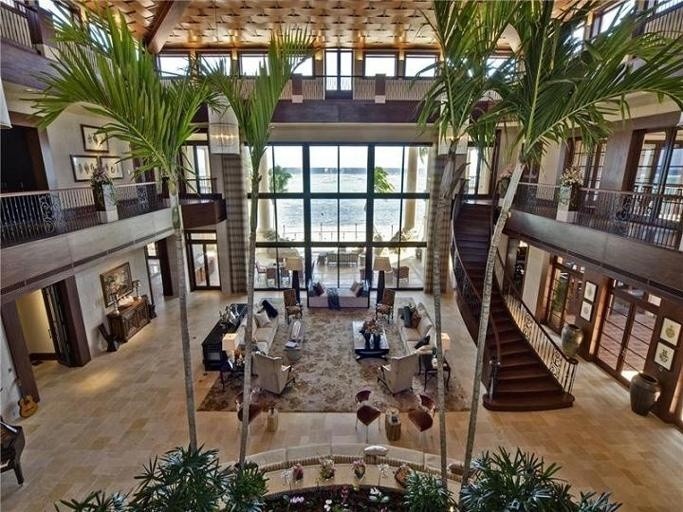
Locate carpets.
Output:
[196,296,470,412]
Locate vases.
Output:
[629,371,662,417]
[560,323,584,359]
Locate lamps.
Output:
[203,0,241,158]
[372,256,391,298]
[286,256,303,303]
[221,333,240,362]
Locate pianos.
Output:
[0,416,25,485]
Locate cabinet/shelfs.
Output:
[106,296,150,342]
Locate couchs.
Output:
[396,301,436,374]
[234,304,279,355]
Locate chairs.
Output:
[353,353,436,447]
[373,288,395,323]
[255,255,290,287]
[234,351,296,434]
[282,288,302,324]
[392,265,410,286]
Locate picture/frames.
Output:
[651,316,682,372]
[98,261,133,307]
[579,280,597,323]
[69,123,124,182]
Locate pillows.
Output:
[414,334,429,350]
[399,305,420,329]
[254,298,278,328]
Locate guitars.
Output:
[14,375,38,418]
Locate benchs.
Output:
[306,278,370,309]
